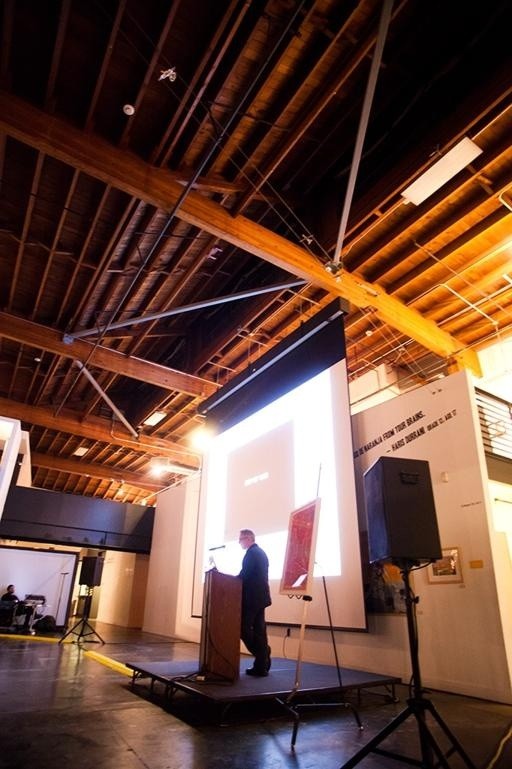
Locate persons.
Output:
[236,530,271,678]
[0,585,18,602]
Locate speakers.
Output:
[79,556,105,586]
[363,456,443,565]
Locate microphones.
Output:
[210,545,226,551]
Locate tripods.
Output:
[59,586,106,650]
[339,565,475,769]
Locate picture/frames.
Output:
[360,533,422,616]
[278,497,322,598]
[427,545,464,584]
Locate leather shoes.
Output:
[265,645,271,672]
[245,666,269,677]
